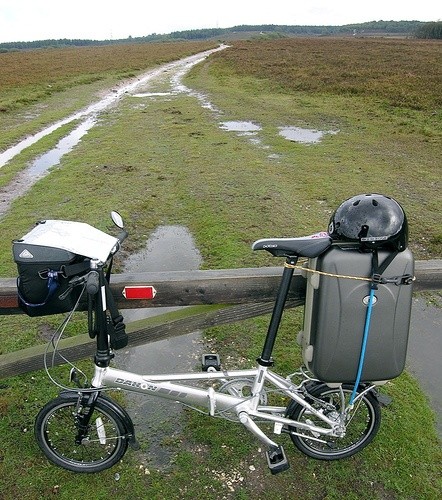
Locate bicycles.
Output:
[13,210,390,475]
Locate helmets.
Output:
[327,193,409,253]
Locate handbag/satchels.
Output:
[13,244,82,317]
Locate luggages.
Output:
[302,247,415,383]
